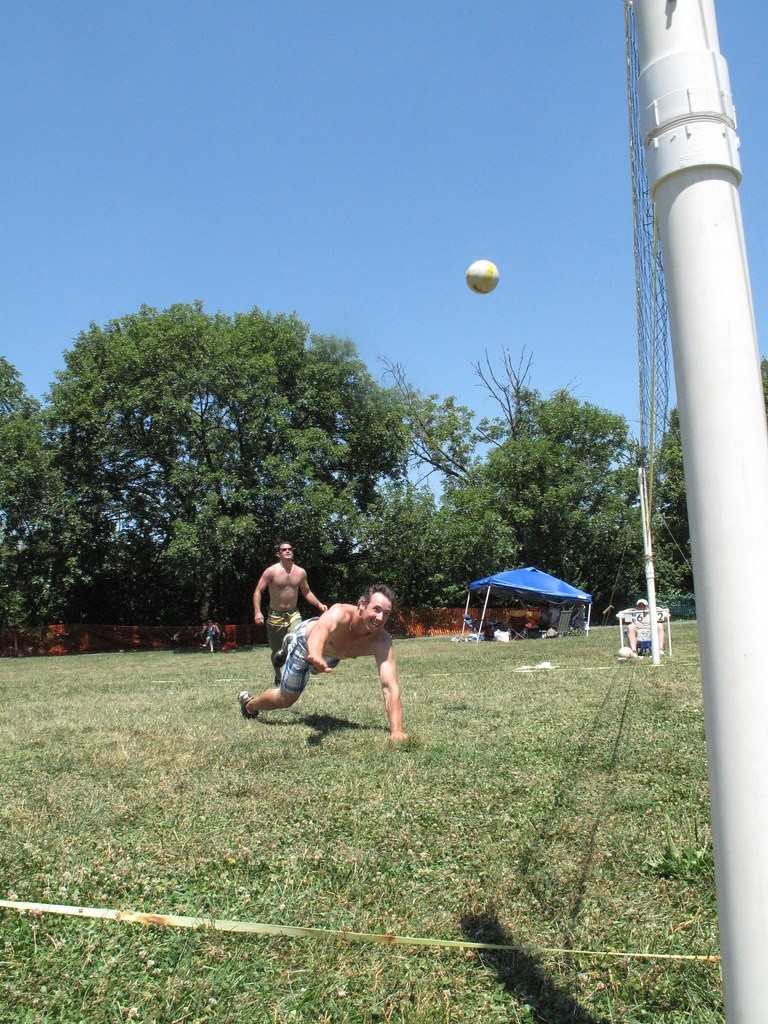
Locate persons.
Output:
[200,619,221,652]
[239,584,409,740]
[253,542,328,686]
[616,599,671,658]
[538,603,560,627]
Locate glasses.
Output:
[279,548,292,551]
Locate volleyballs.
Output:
[466,258,499,294]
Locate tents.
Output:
[461,566,592,642]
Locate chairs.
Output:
[509,614,527,641]
[624,629,652,655]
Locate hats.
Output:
[637,598,648,606]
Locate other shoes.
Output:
[274,634,293,668]
[634,650,639,657]
[275,673,282,686]
[660,649,664,656]
[239,691,259,719]
